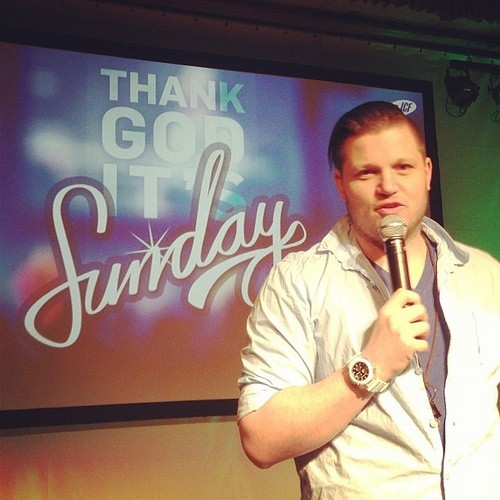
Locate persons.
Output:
[237,101,500,499]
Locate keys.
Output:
[430,398,442,416]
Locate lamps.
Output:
[443,58,481,119]
[486,69,500,123]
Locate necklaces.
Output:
[370,232,438,401]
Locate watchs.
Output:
[345,354,391,392]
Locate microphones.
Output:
[379,214,415,309]
